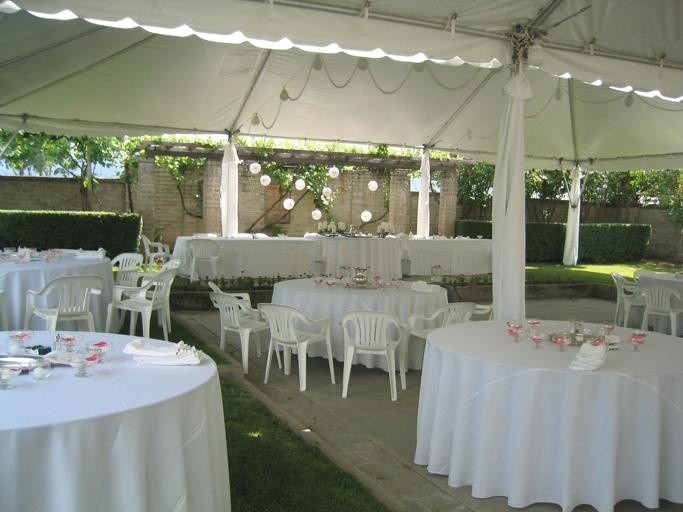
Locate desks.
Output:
[172,235,491,282]
[0,330,230,512]
[1,247,112,330]
[415,319,683,512]
[272,277,448,372]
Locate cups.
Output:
[0,363,24,390]
[28,360,52,380]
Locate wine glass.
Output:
[87,343,112,364]
[625,336,646,351]
[504,321,523,342]
[61,335,81,353]
[526,319,543,329]
[605,324,616,335]
[527,329,548,350]
[632,329,648,341]
[552,333,572,351]
[8,330,33,351]
[69,356,98,377]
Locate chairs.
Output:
[412,301,493,342]
[208,281,270,374]
[25,233,180,342]
[610,270,683,334]
[340,310,407,401]
[258,303,335,392]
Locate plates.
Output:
[0,354,44,371]
